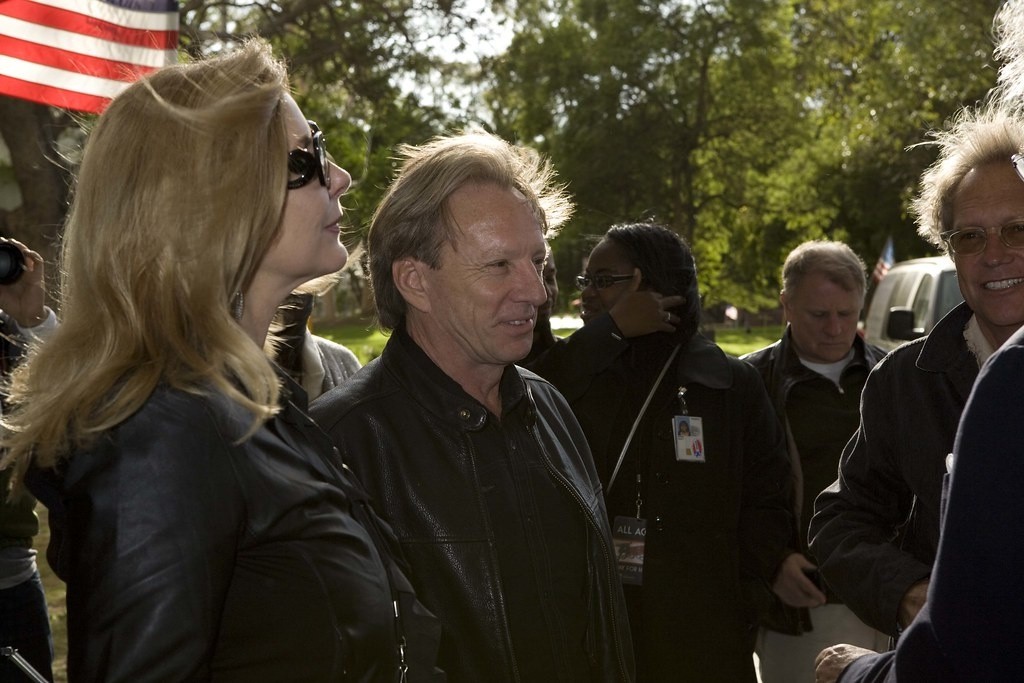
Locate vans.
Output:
[860,254,964,355]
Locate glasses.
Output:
[288,120,329,190]
[575,274,633,290]
[939,217,1024,257]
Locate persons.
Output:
[515,223,801,683]
[815,0,1024,683]
[807,114,1024,682]
[0,43,443,683]
[309,132,637,683]
[679,421,691,437]
[738,241,887,683]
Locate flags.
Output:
[0,0,179,113]
